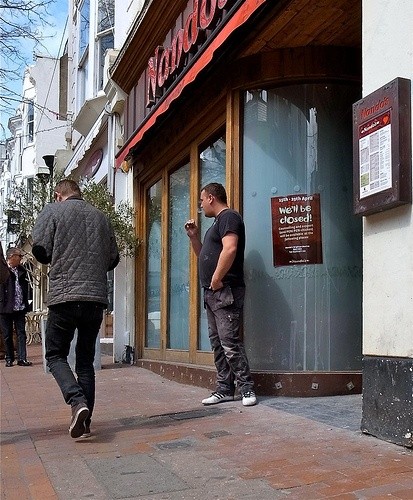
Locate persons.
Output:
[0,240,33,367]
[184,183,258,406]
[31,179,120,439]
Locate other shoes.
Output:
[17,360,32,366]
[5,360,14,367]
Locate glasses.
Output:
[12,254,22,258]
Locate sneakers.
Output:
[69,403,89,438]
[202,391,234,403]
[81,418,91,438]
[241,391,257,406]
